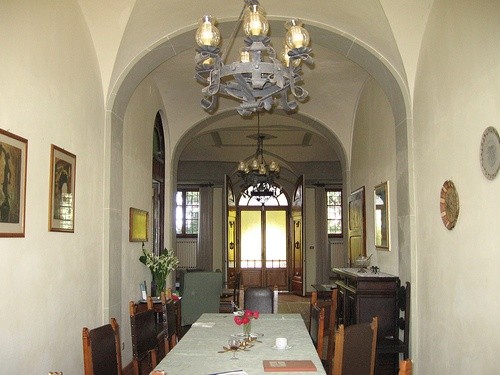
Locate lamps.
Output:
[194,0,315,119]
[236,135,281,186]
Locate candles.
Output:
[276,337,288,348]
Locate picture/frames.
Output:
[0,128,28,239]
[48,143,77,233]
[128,207,149,243]
[373,180,392,252]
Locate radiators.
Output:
[177,239,197,269]
[329,241,344,269]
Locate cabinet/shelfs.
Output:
[332,267,401,375]
[136,296,182,354]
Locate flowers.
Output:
[234,310,260,332]
[138,241,180,291]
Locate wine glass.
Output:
[228,338,240,360]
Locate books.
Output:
[263,360,317,372]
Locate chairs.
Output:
[309,292,325,359]
[180,271,224,328]
[129,296,157,375]
[378,280,411,375]
[82,317,123,375]
[159,288,178,354]
[220,271,242,311]
[238,284,279,314]
[321,315,378,375]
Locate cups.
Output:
[275,338,287,349]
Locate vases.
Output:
[154,280,167,297]
[243,323,251,334]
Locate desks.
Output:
[148,312,327,375]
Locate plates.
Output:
[272,346,290,350]
[478,126,500,181]
[439,180,459,230]
